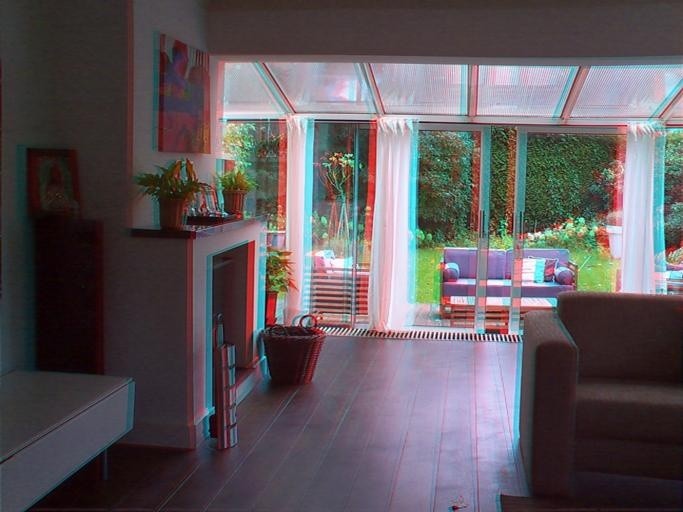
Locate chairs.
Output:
[517,293,683,499]
[304,249,371,315]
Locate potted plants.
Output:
[225,122,257,172]
[218,173,254,213]
[259,206,287,249]
[265,243,299,326]
[131,157,201,231]
[606,210,623,257]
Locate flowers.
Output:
[314,152,363,220]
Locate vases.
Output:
[328,201,349,256]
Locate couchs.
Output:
[439,248,578,319]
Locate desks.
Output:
[0,369,135,512]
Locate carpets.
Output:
[500,494,683,512]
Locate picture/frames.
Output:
[27,148,85,219]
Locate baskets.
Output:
[262,314,327,386]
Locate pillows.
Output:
[443,247,573,285]
[330,256,352,274]
